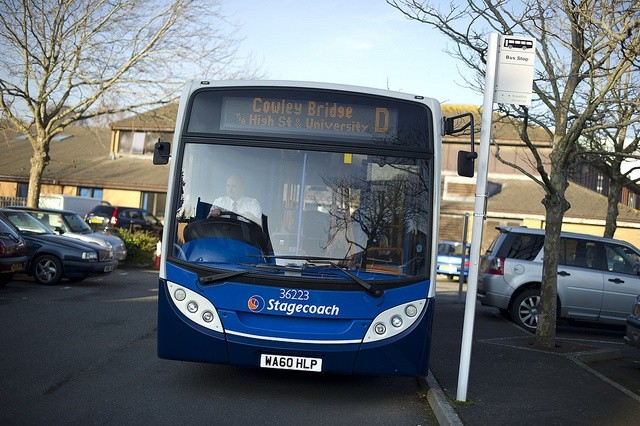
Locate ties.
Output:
[229,202,238,221]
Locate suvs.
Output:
[84,206,163,243]
[477,226,640,334]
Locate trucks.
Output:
[436,240,471,283]
[38,194,112,219]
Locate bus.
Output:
[153,79,478,378]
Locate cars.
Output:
[0,211,28,283]
[5,206,127,265]
[0,209,118,286]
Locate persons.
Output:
[206,175,264,232]
